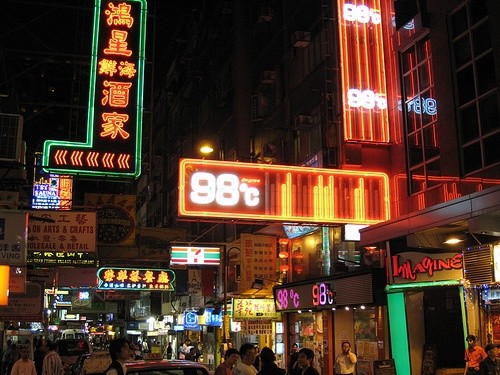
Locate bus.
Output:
[122,360,213,375]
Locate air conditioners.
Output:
[0,113,24,163]
[292,31,310,48]
[263,70,279,83]
[258,6,276,22]
[295,115,313,129]
[262,144,276,158]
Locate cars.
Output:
[70,352,144,375]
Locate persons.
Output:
[463,335,500,375]
[0,336,359,375]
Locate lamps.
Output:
[335,257,365,273]
[251,278,286,289]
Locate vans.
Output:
[54,339,94,375]
[60,330,86,342]
